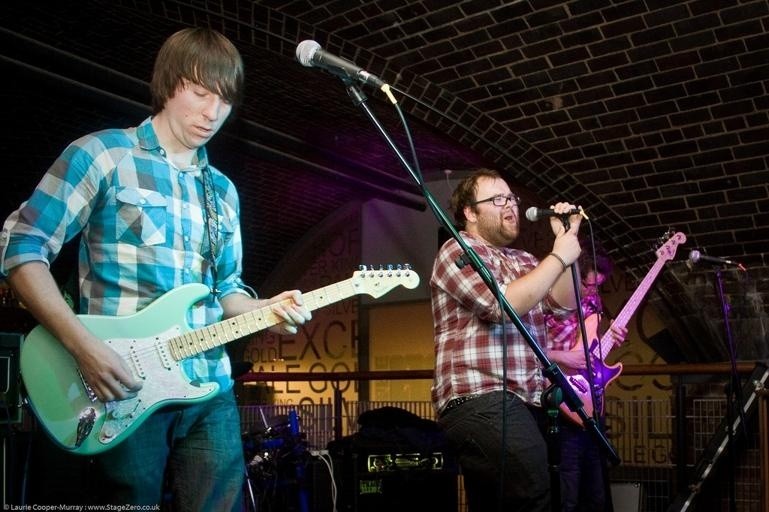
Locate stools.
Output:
[446,445,570,509]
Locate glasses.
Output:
[468,195,522,207]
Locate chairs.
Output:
[327,405,460,512]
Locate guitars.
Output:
[541,224,688,429]
[18,264,421,456]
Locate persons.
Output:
[429,169,628,512]
[0,27,312,512]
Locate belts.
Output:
[445,395,479,413]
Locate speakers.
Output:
[326,431,460,511]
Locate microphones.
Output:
[296,40,389,93]
[525,207,581,222]
[689,250,739,267]
